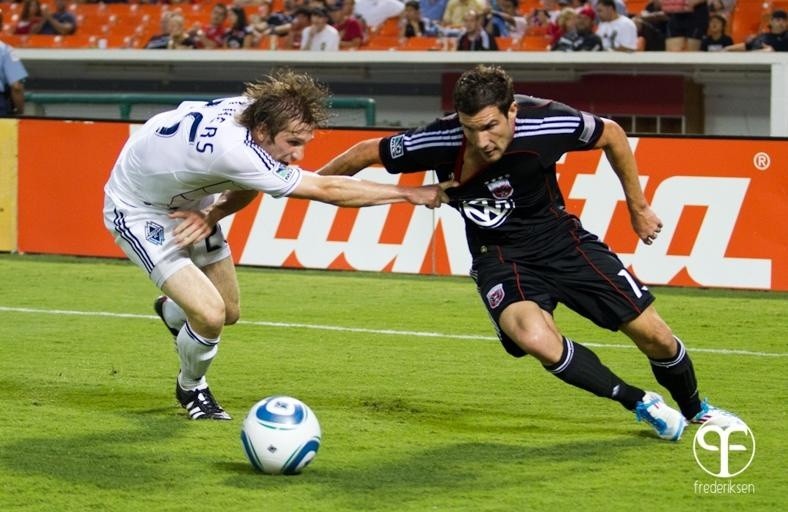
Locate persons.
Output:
[309,60,752,448]
[13,0,44,35]
[0,10,27,116]
[30,0,77,35]
[99,65,460,423]
[140,1,787,50]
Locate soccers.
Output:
[242,396,321,474]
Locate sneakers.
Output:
[174,368,233,422]
[632,389,688,442]
[687,400,746,432]
[151,294,188,356]
